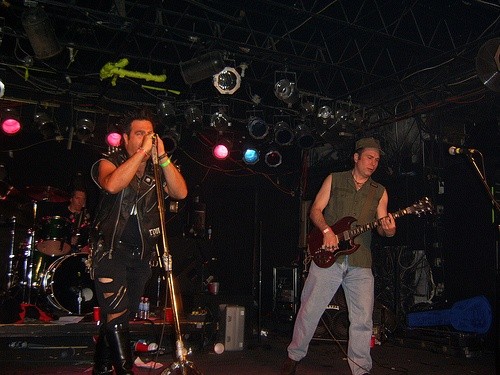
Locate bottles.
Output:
[144,297,150,320]
[138,297,145,318]
[195,185,200,203]
[207,225,212,240]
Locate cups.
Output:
[166,308,173,322]
[135,340,149,353]
[93,306,101,321]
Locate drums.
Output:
[36,215,74,257]
[77,223,91,253]
[38,253,99,316]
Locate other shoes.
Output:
[281,357,298,375]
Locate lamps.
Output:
[0,49,374,168]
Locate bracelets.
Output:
[322,227,331,235]
[157,153,167,160]
[159,158,171,167]
[138,149,151,158]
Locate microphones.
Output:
[0,81,5,97]
[449,147,478,155]
[153,134,157,141]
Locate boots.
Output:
[92,322,112,375]
[106,323,134,375]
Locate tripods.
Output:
[151,140,206,375]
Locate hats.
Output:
[356,137,385,156]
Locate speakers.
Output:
[300,271,352,343]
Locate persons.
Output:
[47,188,93,316]
[280,137,396,375]
[86,108,188,375]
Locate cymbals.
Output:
[23,186,71,202]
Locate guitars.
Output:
[308,197,434,269]
